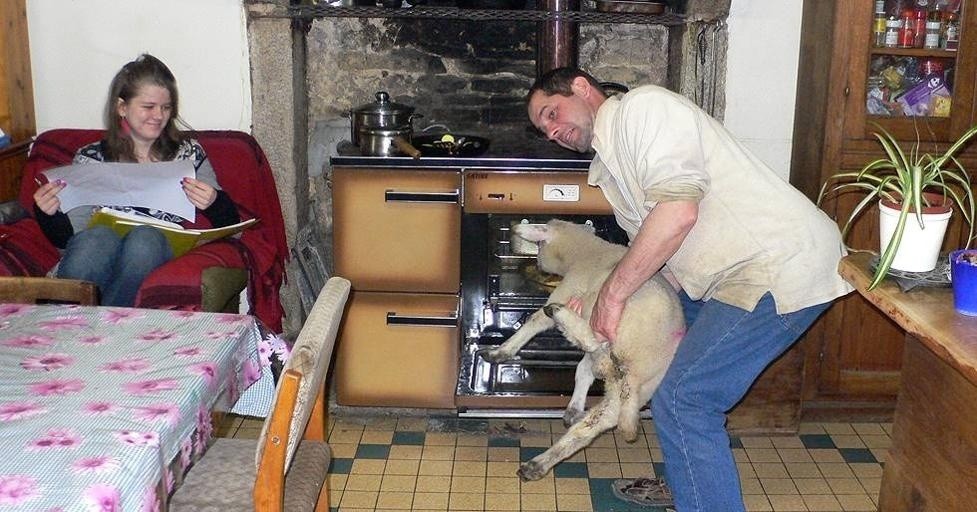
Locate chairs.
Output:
[168,277,354,512]
[0,276,96,309]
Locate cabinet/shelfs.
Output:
[787,1,976,425]
[328,159,461,415]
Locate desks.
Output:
[1,296,292,512]
[837,238,977,511]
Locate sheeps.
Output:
[479,218,686,482]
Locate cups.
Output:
[950,249,977,317]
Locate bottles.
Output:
[872,0,887,48]
[938,11,959,51]
[899,12,913,48]
[885,1,903,48]
[925,1,942,48]
[910,11,926,48]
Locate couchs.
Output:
[0,128,289,315]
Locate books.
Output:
[84,205,262,258]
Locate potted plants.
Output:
[820,116,976,292]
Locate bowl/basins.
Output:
[517,257,557,296]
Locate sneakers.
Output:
[610,476,675,507]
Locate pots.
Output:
[359,126,420,158]
[342,92,414,147]
[413,124,491,157]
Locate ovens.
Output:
[457,174,654,418]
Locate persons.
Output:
[525,67,858,510]
[33,53,239,308]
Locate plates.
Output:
[871,256,953,289]
